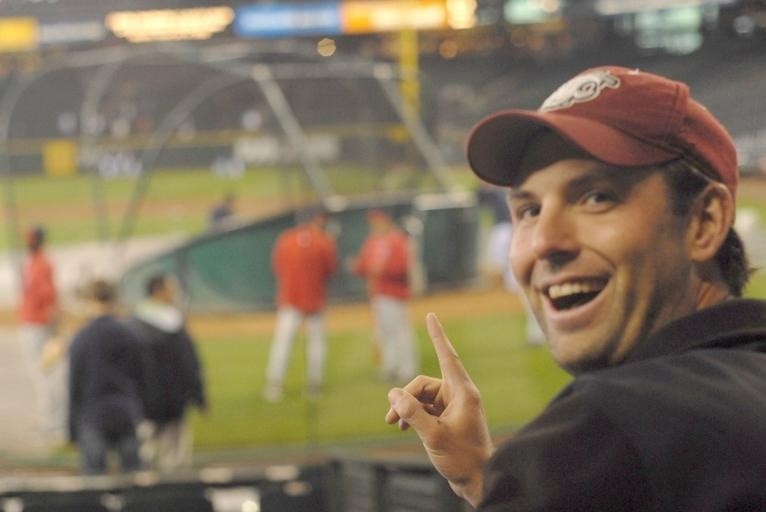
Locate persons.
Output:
[126,270,217,461]
[383,65,764,510]
[256,202,341,402]
[346,203,418,395]
[210,192,238,229]
[20,223,71,444]
[60,279,146,472]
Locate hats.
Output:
[465,64,739,198]
